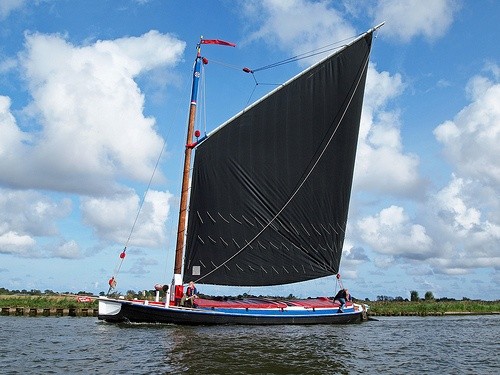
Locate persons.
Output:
[180,281,198,308]
[333,288,349,313]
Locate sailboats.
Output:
[92,20,387,327]
